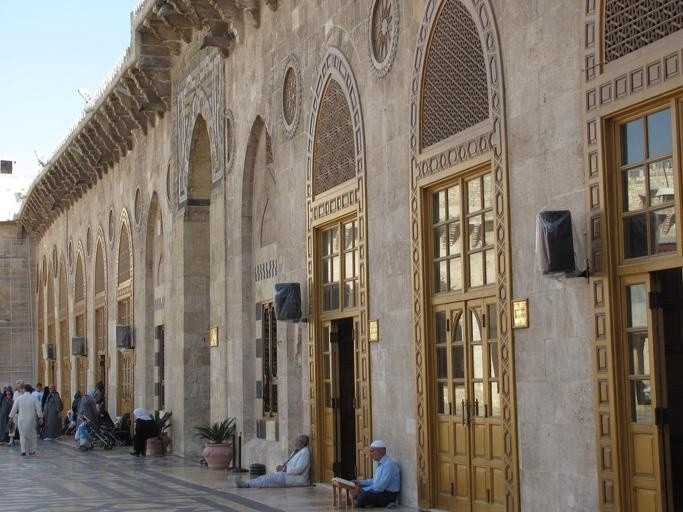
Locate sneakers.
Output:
[43,437,52,440]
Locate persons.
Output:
[0,379,62,456]
[228,434,311,488]
[64,389,105,451]
[350,440,401,507]
[129,408,157,456]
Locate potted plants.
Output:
[192,416,236,470]
[146,411,172,456]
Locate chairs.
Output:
[332,462,357,508]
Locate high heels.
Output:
[130,451,140,456]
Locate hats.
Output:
[370,440,385,447]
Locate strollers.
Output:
[74,413,118,452]
